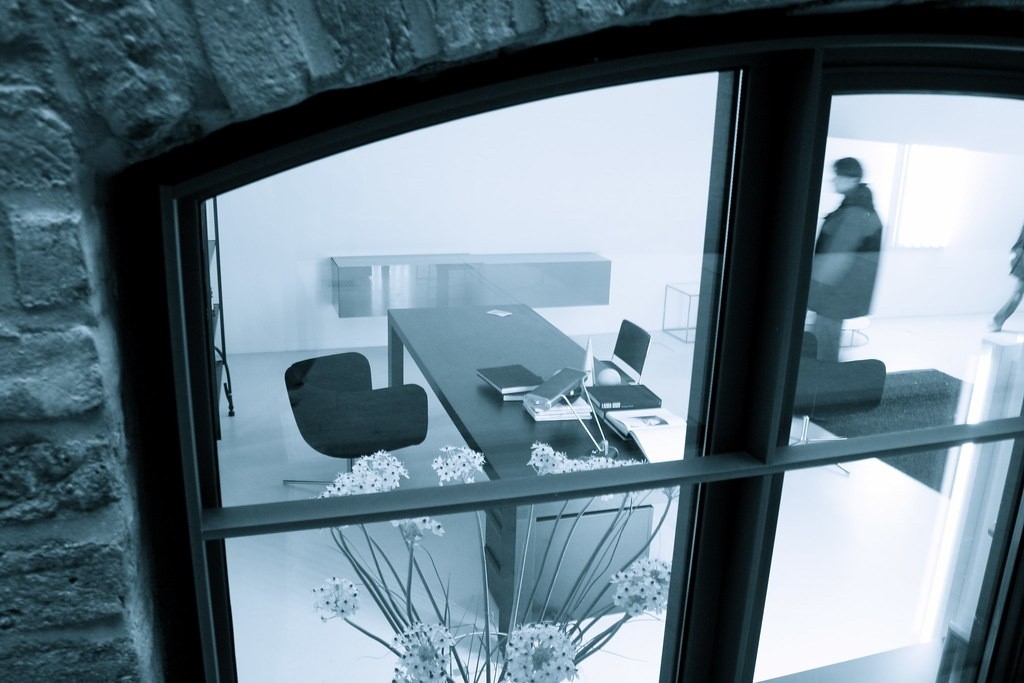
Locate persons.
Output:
[807,158,883,362]
[993,224,1024,330]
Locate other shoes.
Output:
[993,319,1001,331]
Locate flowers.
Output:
[311,439,681,683]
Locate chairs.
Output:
[601,319,652,385]
[282,352,428,486]
[785,332,886,474]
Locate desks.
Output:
[387,304,655,657]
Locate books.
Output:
[477,365,687,463]
[586,385,662,410]
